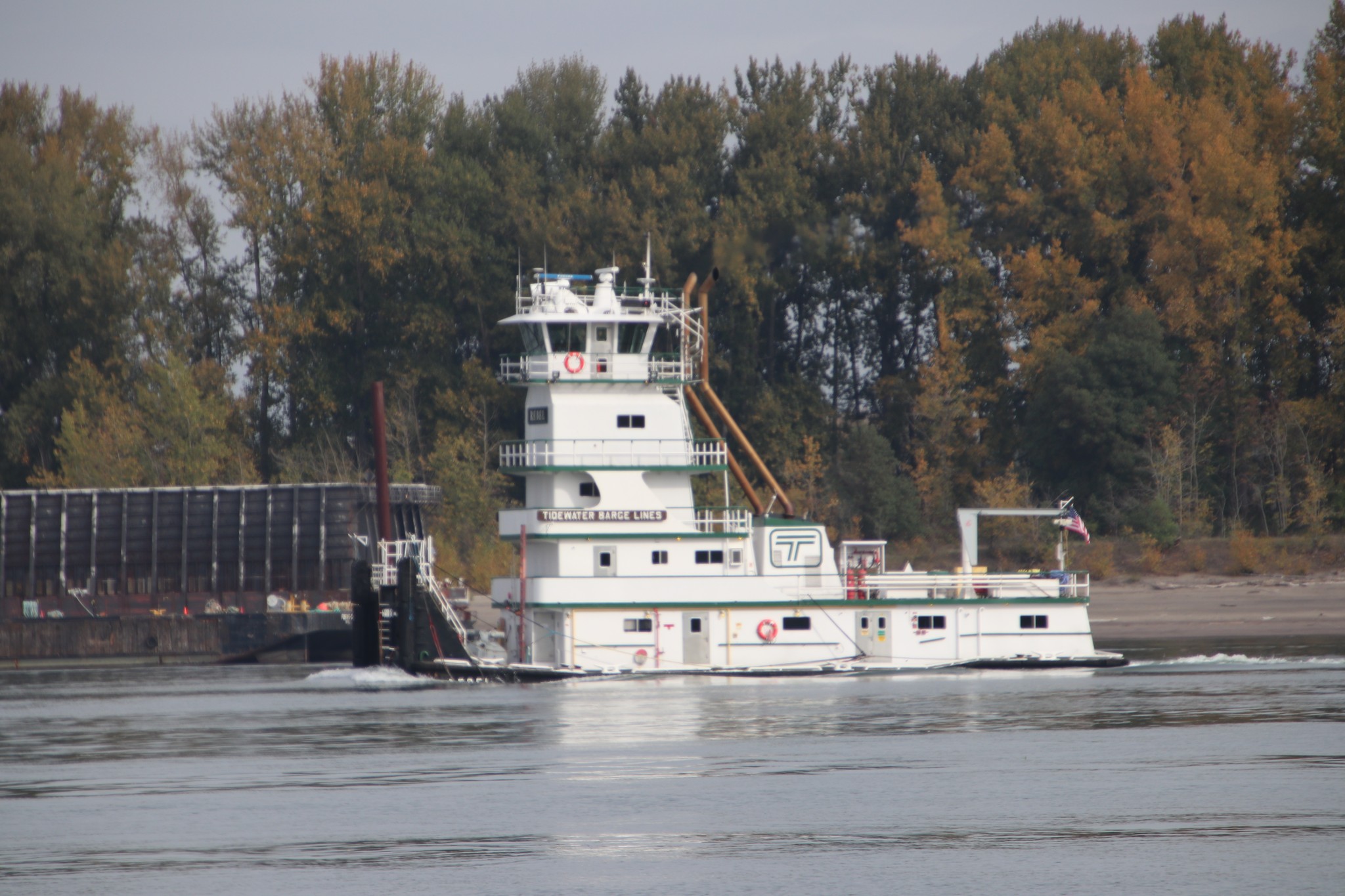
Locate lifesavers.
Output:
[565,351,585,373]
[758,620,777,640]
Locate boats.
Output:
[421,230,1127,686]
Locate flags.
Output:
[1061,504,1091,545]
